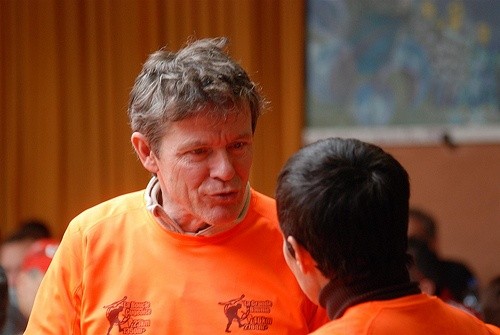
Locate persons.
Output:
[277,136,499,335]
[404,207,500,329]
[0,216,63,335]
[22,36,330,335]
